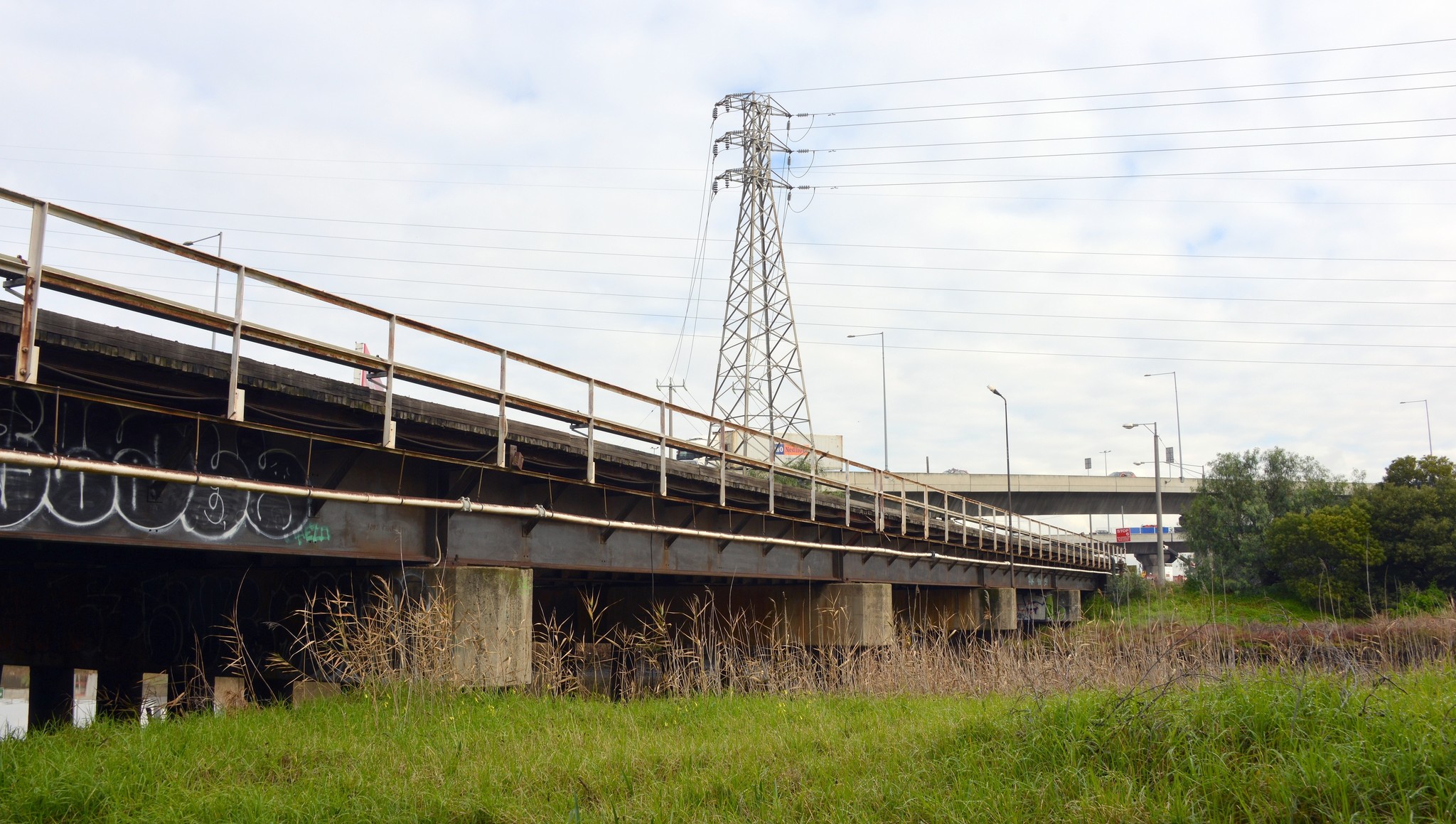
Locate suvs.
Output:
[1108,471,1136,477]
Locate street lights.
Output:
[847,331,891,478]
[1144,372,1184,482]
[1399,399,1432,457]
[182,231,223,351]
[1133,460,1206,494]
[1122,422,1165,584]
[987,385,1015,588]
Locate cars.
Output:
[943,468,970,474]
